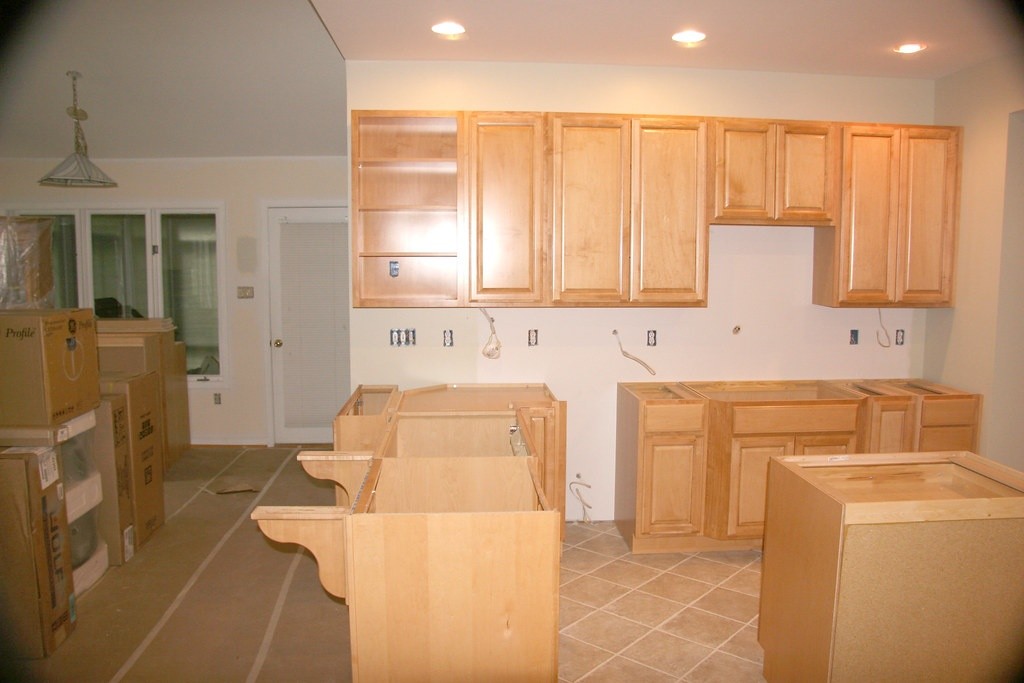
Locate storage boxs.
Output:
[0,308,165,659]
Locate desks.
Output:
[98,320,191,461]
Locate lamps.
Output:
[37,70,119,190]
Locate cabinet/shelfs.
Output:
[813,121,965,308]
[614,379,982,555]
[709,116,843,228]
[351,110,712,309]
[757,450,1024,683]
